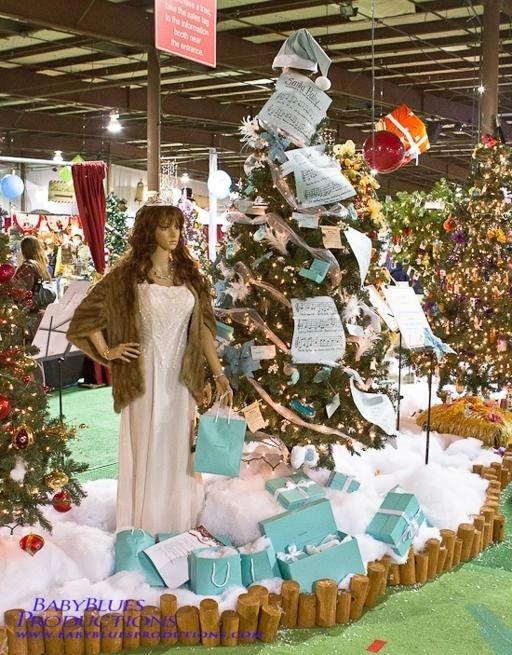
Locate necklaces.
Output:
[147,267,179,282]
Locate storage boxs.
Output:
[365,492,426,557]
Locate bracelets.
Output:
[102,344,112,362]
[210,372,226,381]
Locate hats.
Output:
[272,28,332,91]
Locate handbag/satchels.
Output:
[116,528,284,596]
[17,280,55,314]
[194,393,246,479]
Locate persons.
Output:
[42,231,92,279]
[11,233,56,340]
[67,184,234,538]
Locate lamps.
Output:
[107,115,121,133]
[181,173,189,181]
[53,151,64,162]
